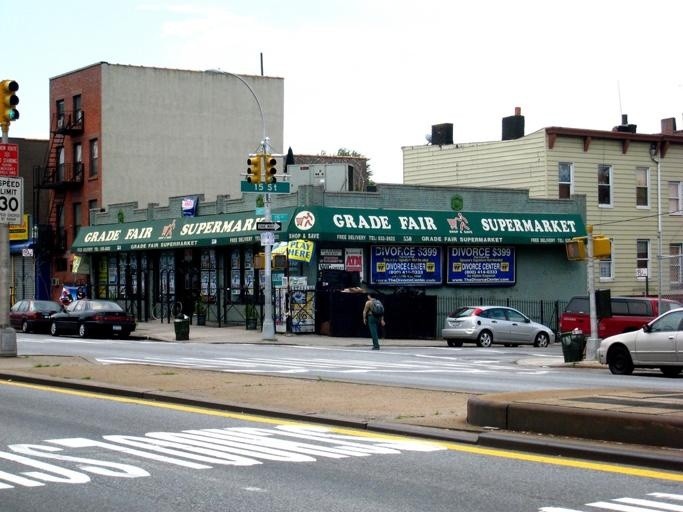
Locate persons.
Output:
[362,292,385,350]
[59,291,72,314]
[76,286,84,301]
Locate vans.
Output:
[560,295,682,363]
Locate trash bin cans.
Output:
[174,318,189,340]
[561,331,587,362]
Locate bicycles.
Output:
[151,297,182,321]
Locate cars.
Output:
[442,306,555,347]
[597,307,683,375]
[48,299,136,338]
[9,300,62,333]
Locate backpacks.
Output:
[368,300,384,318]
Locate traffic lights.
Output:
[564,240,585,260]
[266,158,278,186]
[0,79,19,128]
[247,157,261,184]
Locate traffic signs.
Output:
[0,178,23,227]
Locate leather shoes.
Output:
[372,344,380,350]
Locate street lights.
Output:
[203,68,277,342]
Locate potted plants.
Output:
[243,303,258,329]
[194,290,210,325]
